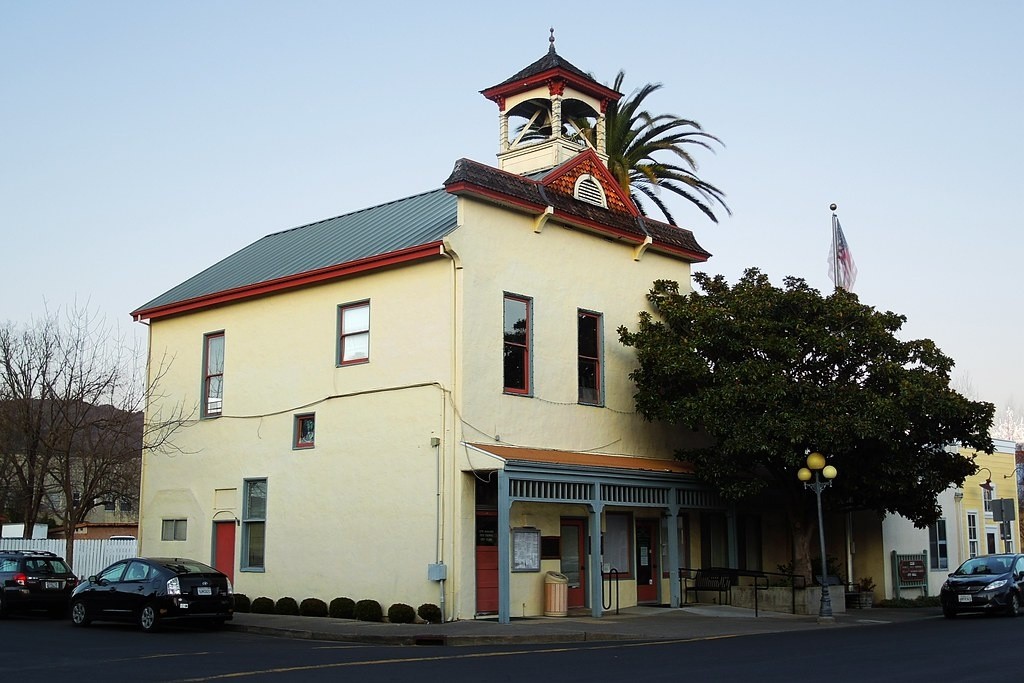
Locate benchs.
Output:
[684,568,735,605]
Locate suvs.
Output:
[0,549,80,619]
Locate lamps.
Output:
[973,468,993,491]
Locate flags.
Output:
[827,215,858,293]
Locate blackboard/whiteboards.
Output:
[510,529,542,573]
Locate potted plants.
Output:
[855,577,876,608]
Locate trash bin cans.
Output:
[544,570,570,617]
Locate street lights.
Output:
[797,452,838,625]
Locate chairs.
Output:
[133,566,145,579]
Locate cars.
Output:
[941,553,1024,616]
[69,556,235,632]
